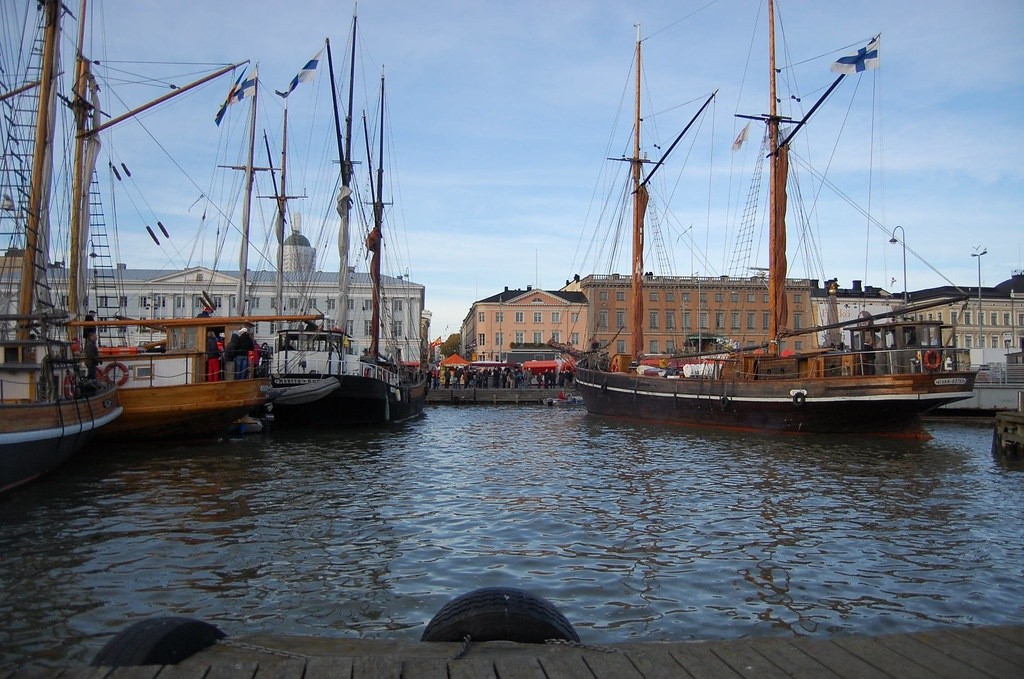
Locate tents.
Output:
[441,354,469,367]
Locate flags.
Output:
[275,45,324,98]
[215,62,259,127]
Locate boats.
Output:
[540,393,583,407]
[991,409,1024,461]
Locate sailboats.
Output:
[254,0,430,433]
[0,1,327,501]
[547,0,981,442]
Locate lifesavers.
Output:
[104,362,129,386]
[924,349,940,369]
[364,367,368,377]
[793,392,805,407]
[89,615,230,663]
[329,327,343,331]
[421,586,581,641]
[96,367,103,383]
[610,364,618,372]
[719,397,729,409]
[601,383,607,391]
[65,372,75,400]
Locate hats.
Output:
[89,310,97,313]
[205,306,214,312]
[238,327,247,332]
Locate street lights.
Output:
[970,250,989,305]
[498,303,504,363]
[889,226,907,319]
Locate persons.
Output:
[863,336,876,375]
[907,331,921,345]
[207,327,272,382]
[196,306,213,318]
[83,310,103,380]
[559,391,564,399]
[281,339,295,351]
[414,366,574,389]
